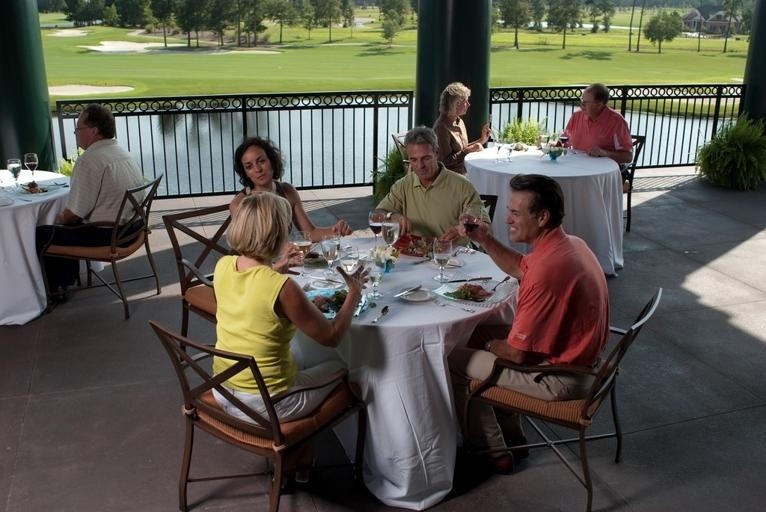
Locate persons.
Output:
[446,176,610,474]
[368,125,491,250]
[229,136,352,262]
[550,84,633,184]
[432,81,492,174]
[211,193,371,486]
[35,105,146,306]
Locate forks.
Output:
[489,276,510,294]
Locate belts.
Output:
[548,361,603,371]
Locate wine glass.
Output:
[541,129,551,156]
[493,138,517,165]
[433,240,453,282]
[369,211,402,269]
[462,204,482,255]
[366,254,386,298]
[322,234,342,277]
[25,152,39,185]
[338,245,361,277]
[293,230,314,279]
[559,130,574,162]
[7,158,24,191]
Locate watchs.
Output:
[386,210,393,222]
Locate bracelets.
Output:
[483,336,492,352]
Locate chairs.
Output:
[33,171,165,322]
[613,132,650,234]
[453,285,668,512]
[460,190,500,255]
[145,316,371,511]
[391,127,416,178]
[157,201,238,365]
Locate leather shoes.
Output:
[476,435,530,478]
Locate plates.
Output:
[444,258,464,268]
[400,286,434,301]
[434,277,513,305]
[309,278,343,290]
[304,290,369,318]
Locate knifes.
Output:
[393,283,423,300]
[373,305,389,325]
[445,277,492,283]
